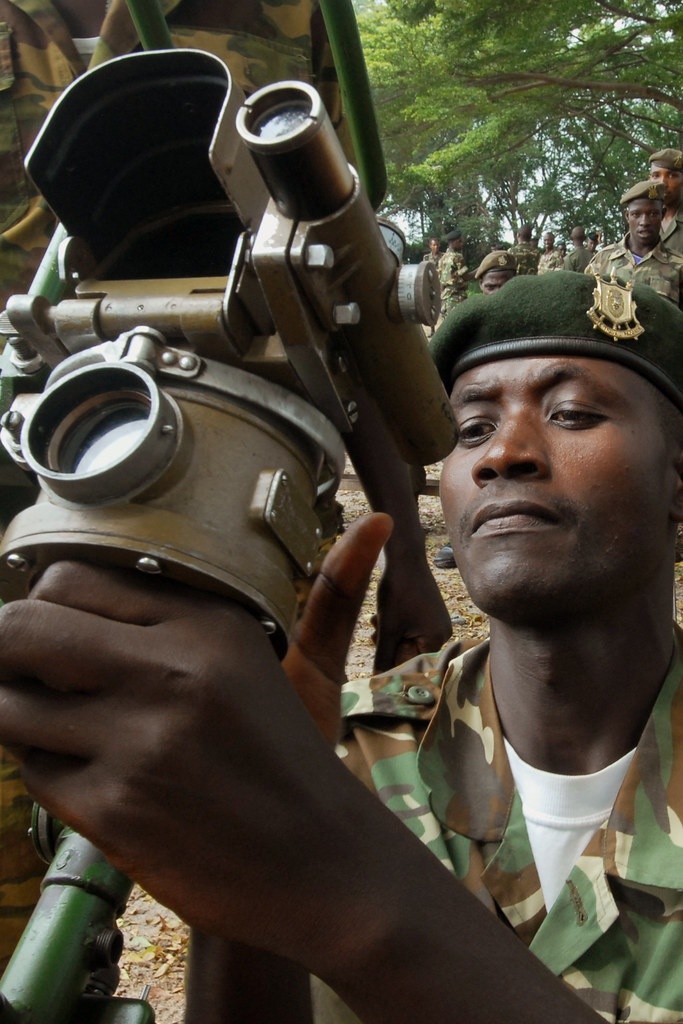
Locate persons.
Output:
[0,270,683,1023]
[585,147,683,314]
[422,225,598,339]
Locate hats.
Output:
[588,233,598,241]
[620,181,664,208]
[446,231,461,242]
[649,148,683,172]
[475,251,517,278]
[428,270,683,415]
